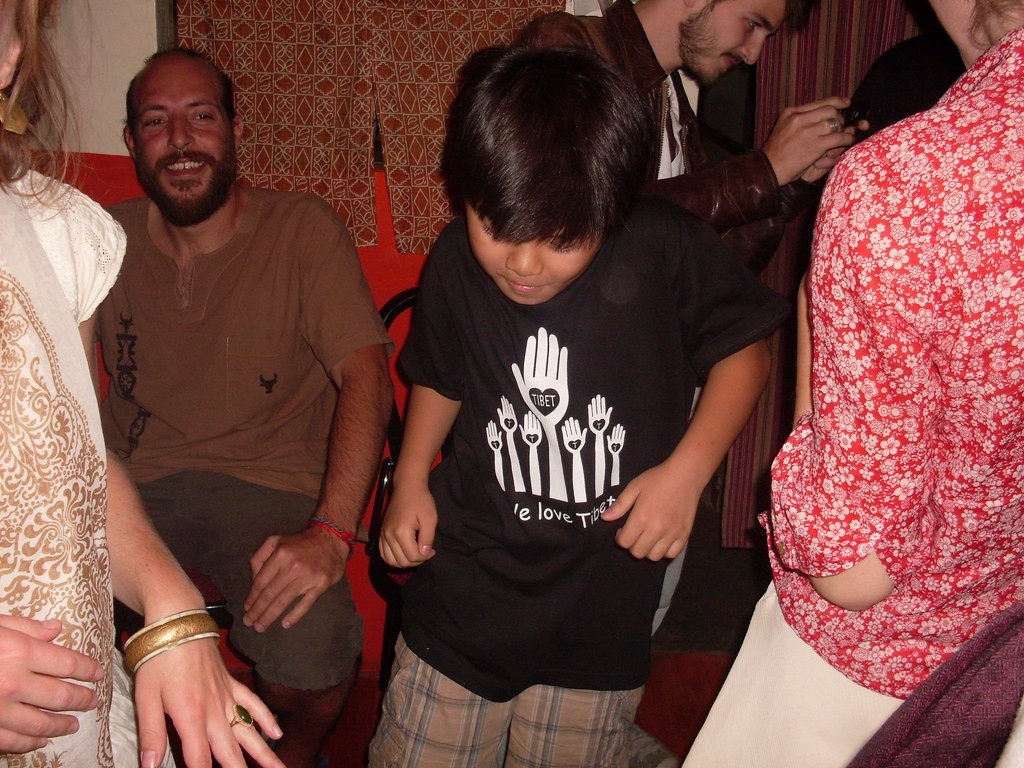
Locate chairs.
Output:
[365,285,702,693]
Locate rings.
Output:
[828,118,838,132]
[230,703,253,728]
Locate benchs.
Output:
[24,149,426,681]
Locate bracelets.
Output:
[309,517,356,556]
[123,610,221,675]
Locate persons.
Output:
[371,46,791,768]
[578,0,871,636]
[682,0,1024,768]
[0,0,283,768]
[76,49,398,768]
